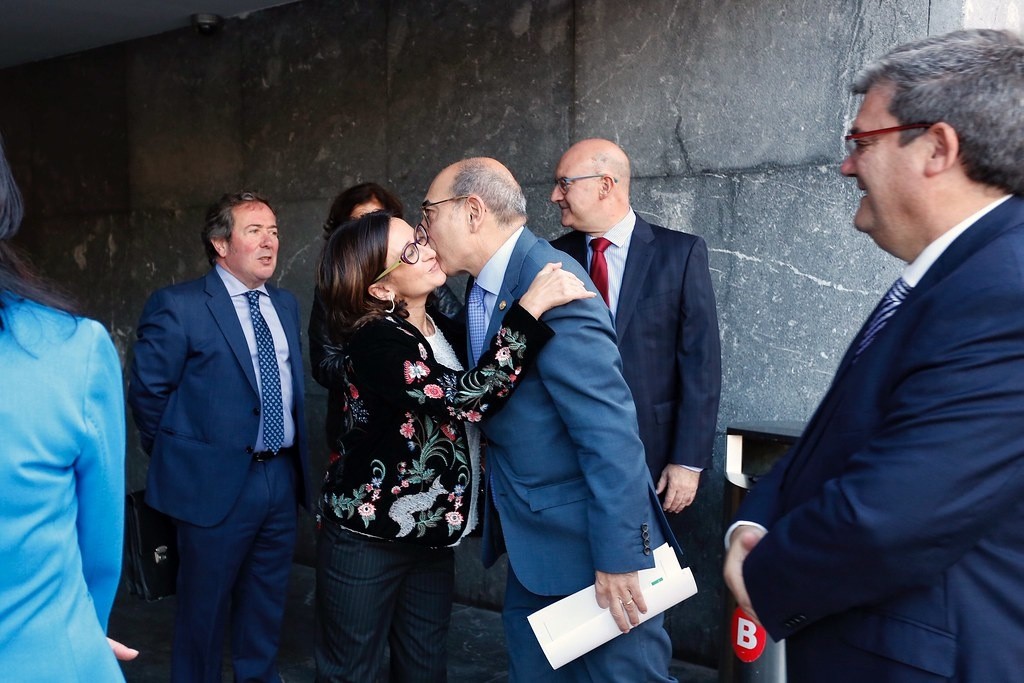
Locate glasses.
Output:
[843,115,960,162]
[420,196,487,228]
[554,173,618,195]
[371,223,429,284]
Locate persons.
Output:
[126,192,312,683]
[0,141,141,683]
[309,138,722,683]
[724,27,1024,683]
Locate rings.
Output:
[622,599,633,606]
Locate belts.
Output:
[252,448,295,462]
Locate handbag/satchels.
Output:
[118,489,179,602]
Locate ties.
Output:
[468,283,499,512]
[854,278,911,358]
[240,290,285,456]
[588,237,611,308]
